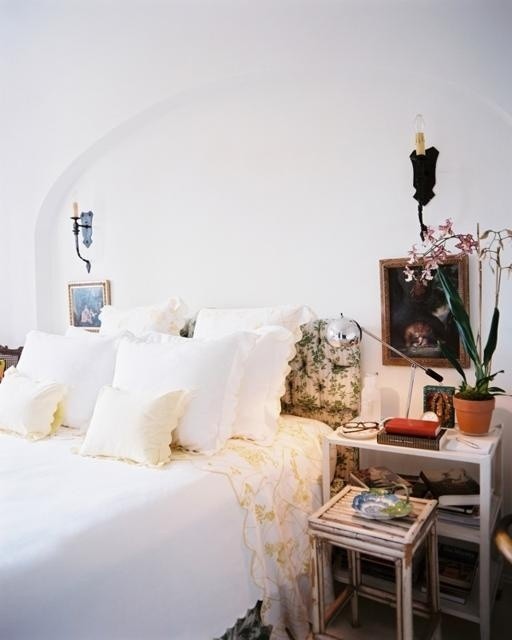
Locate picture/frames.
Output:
[380,254,470,369]
[68,280,109,332]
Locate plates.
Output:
[339,423,382,440]
[351,484,413,523]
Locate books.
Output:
[377,429,447,451]
[423,384,455,428]
[385,418,442,438]
[350,464,414,494]
[437,505,475,514]
[421,466,480,505]
[420,540,480,606]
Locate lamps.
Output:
[409,113,438,241]
[326,313,443,420]
[69,190,92,273]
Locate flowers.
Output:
[403,214,512,399]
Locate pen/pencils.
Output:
[456,436,480,449]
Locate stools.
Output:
[308,485,443,640]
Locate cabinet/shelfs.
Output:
[321,416,504,640]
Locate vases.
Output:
[453,391,495,436]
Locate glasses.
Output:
[342,422,381,433]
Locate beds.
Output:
[0,318,362,640]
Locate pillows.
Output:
[76,381,193,465]
[0,362,77,443]
[15,306,306,452]
